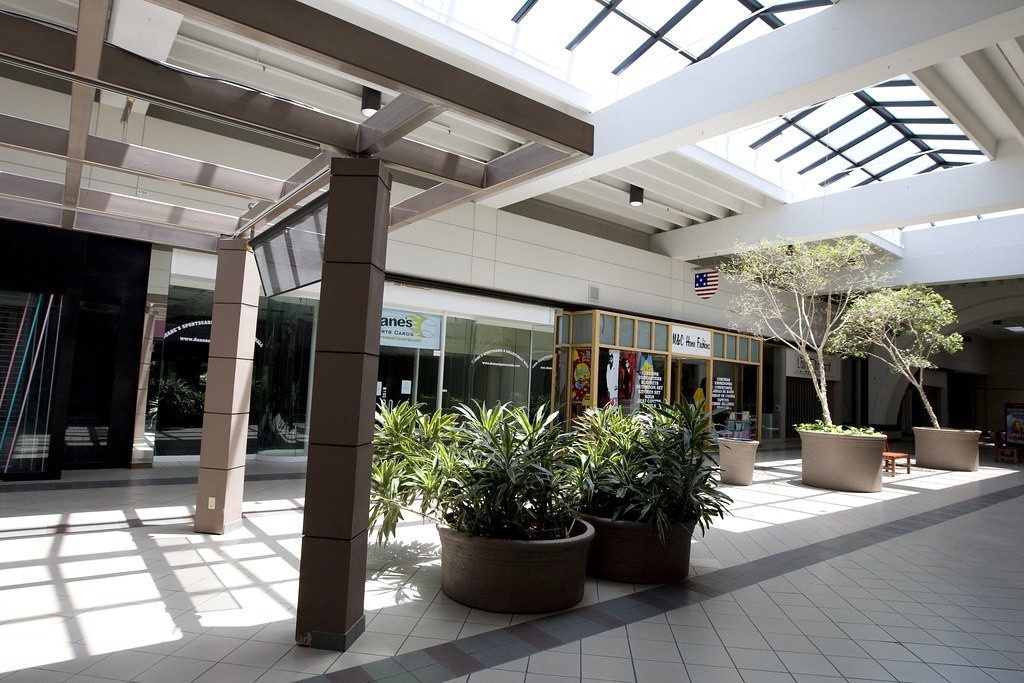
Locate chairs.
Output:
[990,431,1022,464]
[979,445,981,462]
[883,440,910,477]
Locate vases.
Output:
[716,437,761,485]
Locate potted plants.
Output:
[148,377,186,428]
[824,279,982,472]
[365,396,595,615]
[572,391,734,585]
[716,234,903,493]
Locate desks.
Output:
[293,423,305,456]
